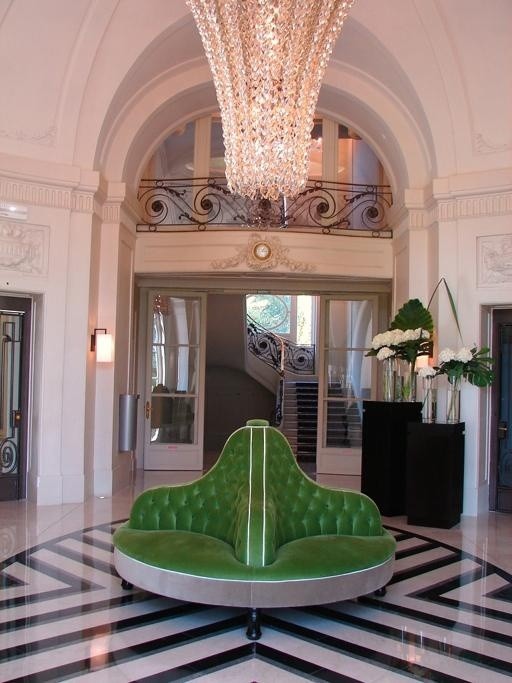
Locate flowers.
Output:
[433,342,497,420]
[364,298,433,395]
[418,365,437,408]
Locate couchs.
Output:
[113,419,397,640]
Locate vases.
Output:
[382,357,417,402]
[446,375,461,425]
[423,375,437,419]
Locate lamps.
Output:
[91,329,112,363]
[185,0,358,200]
